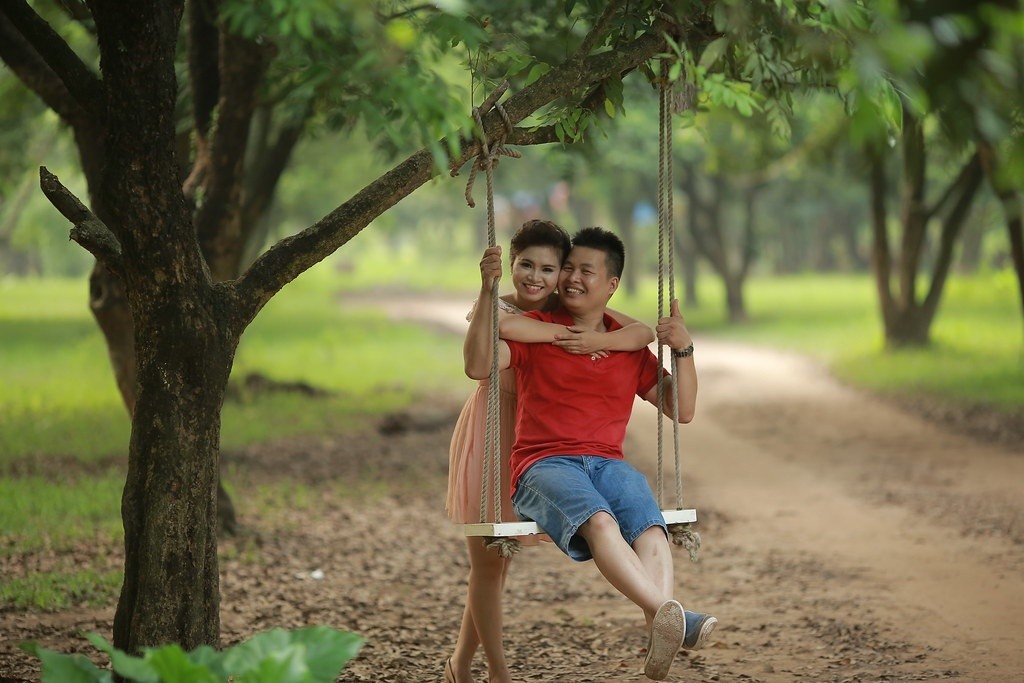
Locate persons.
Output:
[462,227,718,679]
[445,219,655,683]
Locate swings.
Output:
[453,58,696,536]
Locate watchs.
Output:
[676,342,693,358]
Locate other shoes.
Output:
[444,657,475,683]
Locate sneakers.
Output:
[642,601,718,678]
